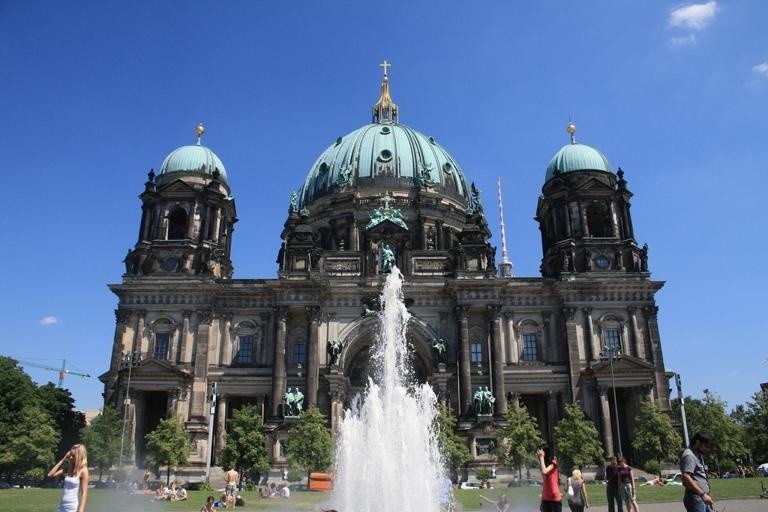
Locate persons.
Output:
[605,456,623,512]
[200,463,245,512]
[618,456,639,512]
[276,241,293,271]
[47,444,89,512]
[436,338,446,355]
[123,467,188,502]
[536,443,563,512]
[564,469,588,512]
[381,242,396,270]
[440,479,456,512]
[479,494,510,512]
[330,340,340,355]
[474,386,492,414]
[258,481,290,499]
[680,431,715,512]
[282,387,304,416]
[453,242,467,268]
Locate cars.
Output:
[507,478,543,486]
[663,472,682,485]
[460,482,494,490]
[708,463,768,479]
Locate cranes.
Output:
[14,356,92,388]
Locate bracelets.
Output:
[701,491,705,497]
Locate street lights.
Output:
[114,349,144,480]
[600,344,628,458]
[703,388,721,478]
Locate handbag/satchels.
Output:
[567,477,574,501]
